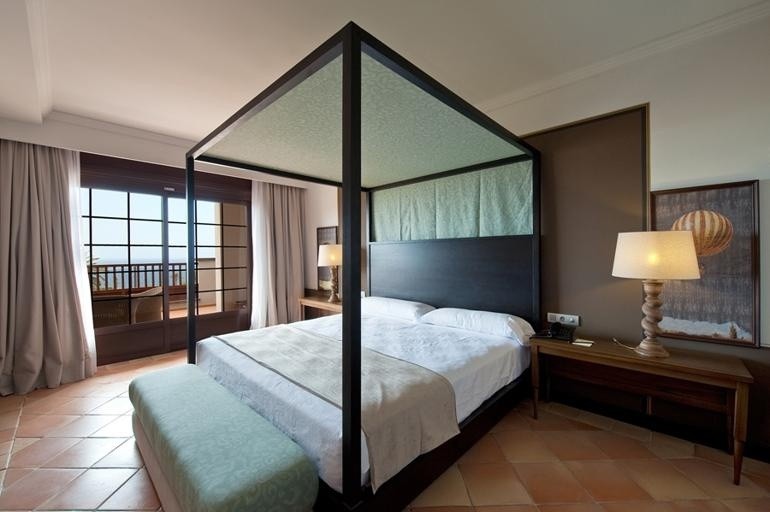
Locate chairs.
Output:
[128,286,164,325]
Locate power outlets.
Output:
[547,311,581,328]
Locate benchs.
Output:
[128,362,321,511]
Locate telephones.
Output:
[551,321,576,341]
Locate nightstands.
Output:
[298,295,343,320]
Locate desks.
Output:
[527,326,755,485]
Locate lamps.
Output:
[318,244,344,303]
[611,229,701,358]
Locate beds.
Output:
[192,238,544,509]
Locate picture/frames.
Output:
[316,226,340,283]
[648,177,762,350]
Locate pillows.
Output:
[362,293,536,348]
